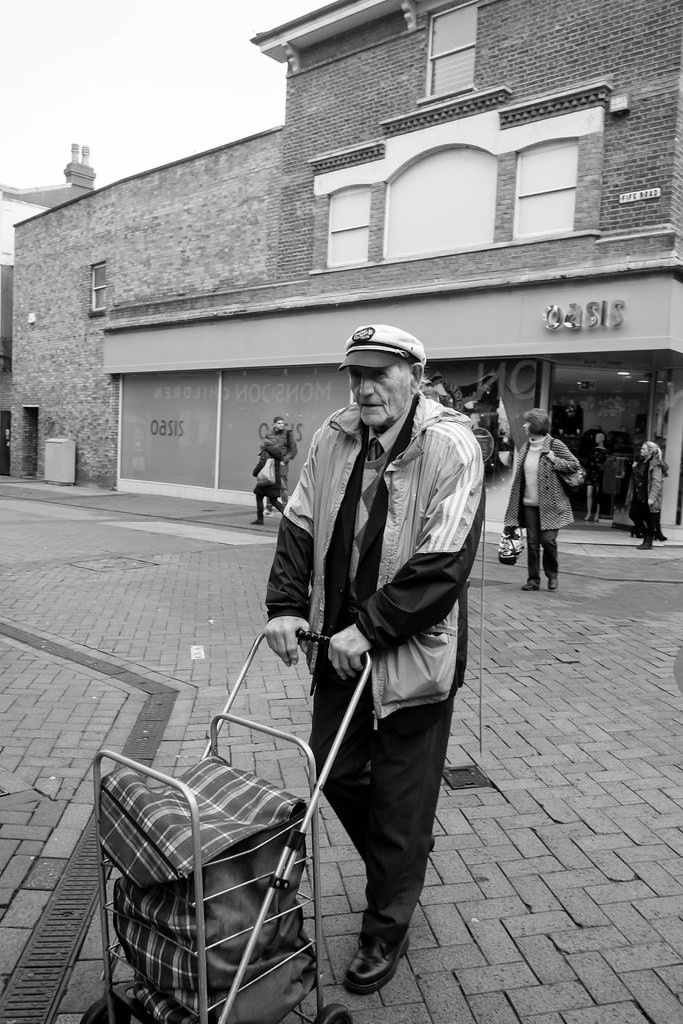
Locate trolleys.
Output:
[81,629,373,1024]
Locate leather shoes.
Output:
[344,928,409,992]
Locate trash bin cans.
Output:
[45,438,76,486]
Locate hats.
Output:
[338,325,426,370]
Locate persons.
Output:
[517,408,581,591]
[266,324,485,993]
[250,417,297,526]
[558,399,581,445]
[628,440,667,549]
[584,433,607,521]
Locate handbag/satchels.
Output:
[498,526,525,565]
[559,465,586,487]
[257,458,276,486]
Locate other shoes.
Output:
[250,519,264,526]
[521,578,541,591]
[548,577,558,590]
[637,543,653,549]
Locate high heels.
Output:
[631,526,644,538]
[654,531,667,541]
